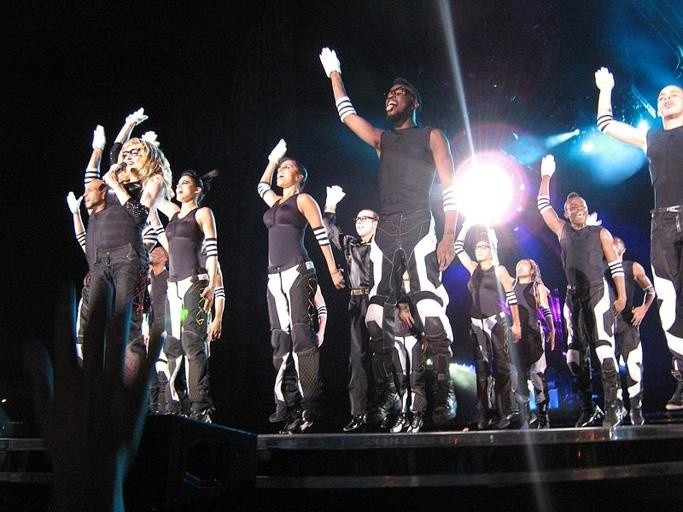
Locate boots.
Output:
[432,380,457,423]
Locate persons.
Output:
[594,65,683,412]
[536,153,627,429]
[69,45,558,434]
[586,210,655,427]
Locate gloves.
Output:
[91,124,107,150]
[594,66,616,135]
[585,212,602,228]
[324,185,347,210]
[320,46,343,78]
[462,215,475,230]
[142,130,161,146]
[267,138,288,166]
[125,108,150,126]
[67,191,82,215]
[540,154,557,179]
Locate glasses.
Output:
[382,85,417,99]
[351,215,378,223]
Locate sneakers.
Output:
[575,400,645,427]
[374,392,423,433]
[146,409,212,424]
[342,406,377,431]
[478,409,550,429]
[666,368,683,410]
[268,404,324,434]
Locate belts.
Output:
[350,289,372,295]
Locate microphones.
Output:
[99,162,127,191]
